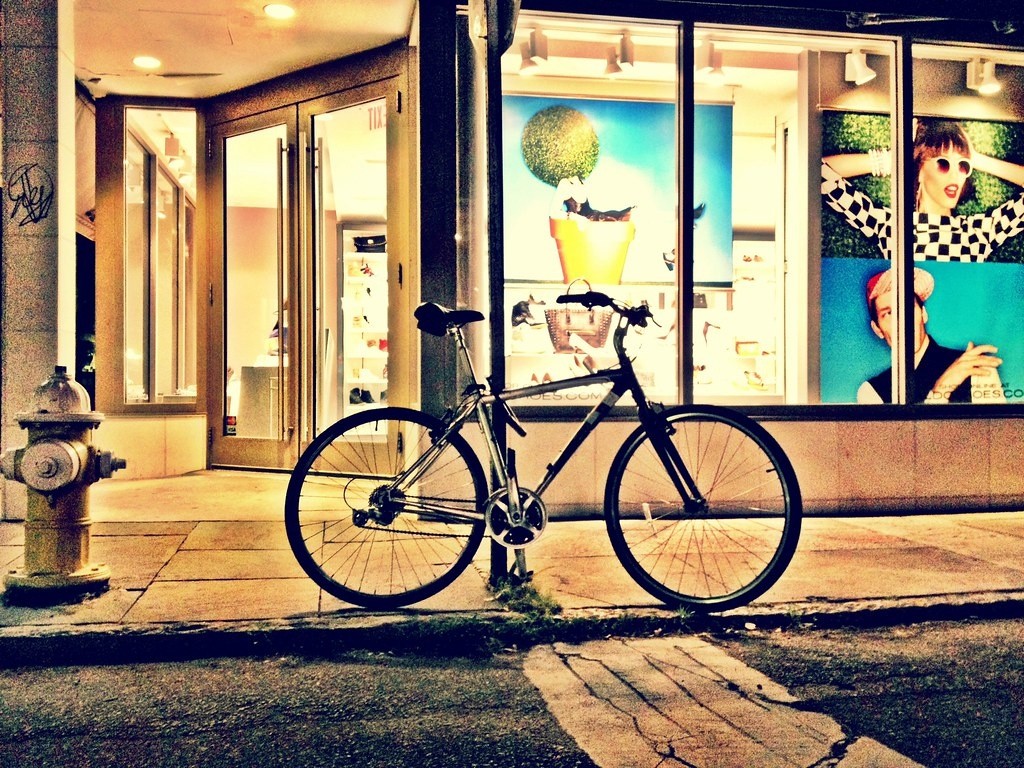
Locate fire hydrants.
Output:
[0,364,127,593]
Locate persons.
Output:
[819,118,1024,262]
[857,266,1008,404]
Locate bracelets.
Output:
[869,148,891,177]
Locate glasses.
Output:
[923,156,971,175]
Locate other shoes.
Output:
[268,256,388,405]
[512,301,546,329]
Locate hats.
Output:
[866,266,934,315]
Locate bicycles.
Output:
[283,291,804,612]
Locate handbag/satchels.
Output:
[544,279,614,352]
[353,235,386,253]
